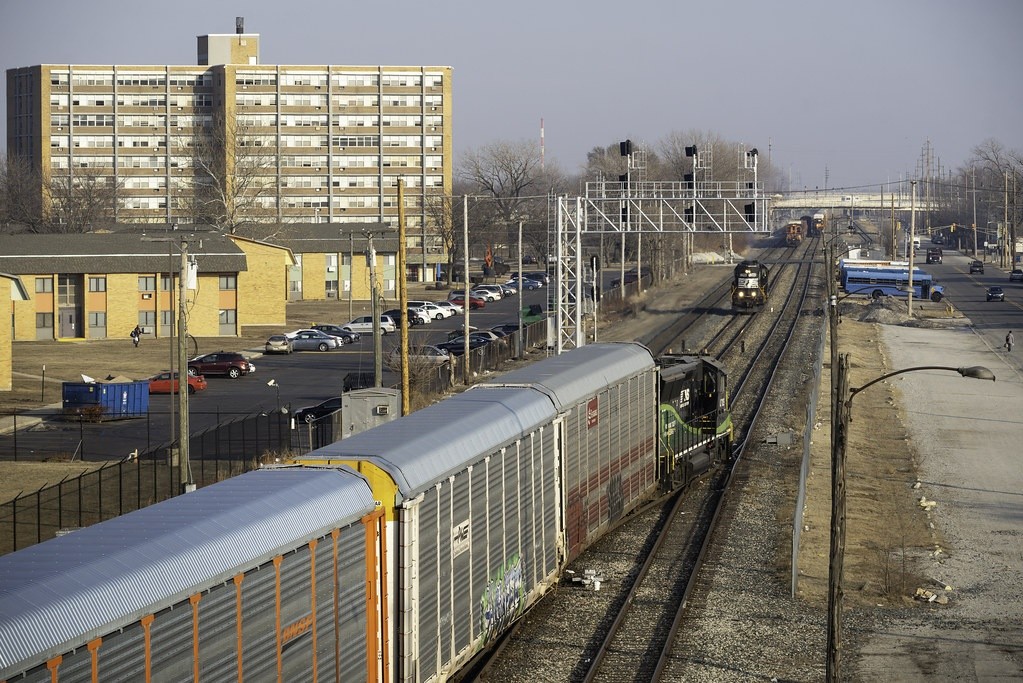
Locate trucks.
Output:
[907,237,921,251]
[926,247,943,263]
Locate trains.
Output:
[1,339,737,682]
[810,209,829,237]
[782,214,811,248]
[728,257,770,315]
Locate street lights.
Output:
[826,287,915,487]
[824,346,1000,683]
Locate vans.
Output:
[389,344,458,372]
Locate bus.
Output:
[841,268,945,302]
[837,258,921,290]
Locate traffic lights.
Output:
[971,225,974,231]
[953,223,956,231]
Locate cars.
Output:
[146,372,208,396]
[930,235,944,245]
[294,395,342,426]
[522,255,539,264]
[494,257,505,264]
[968,261,984,274]
[1009,269,1023,282]
[290,271,552,355]
[264,335,293,354]
[542,253,555,263]
[986,286,1004,302]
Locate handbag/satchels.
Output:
[1004,344,1008,347]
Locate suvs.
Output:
[612,266,651,288]
[188,351,251,379]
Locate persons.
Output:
[1006,331,1013,352]
[133,325,143,347]
